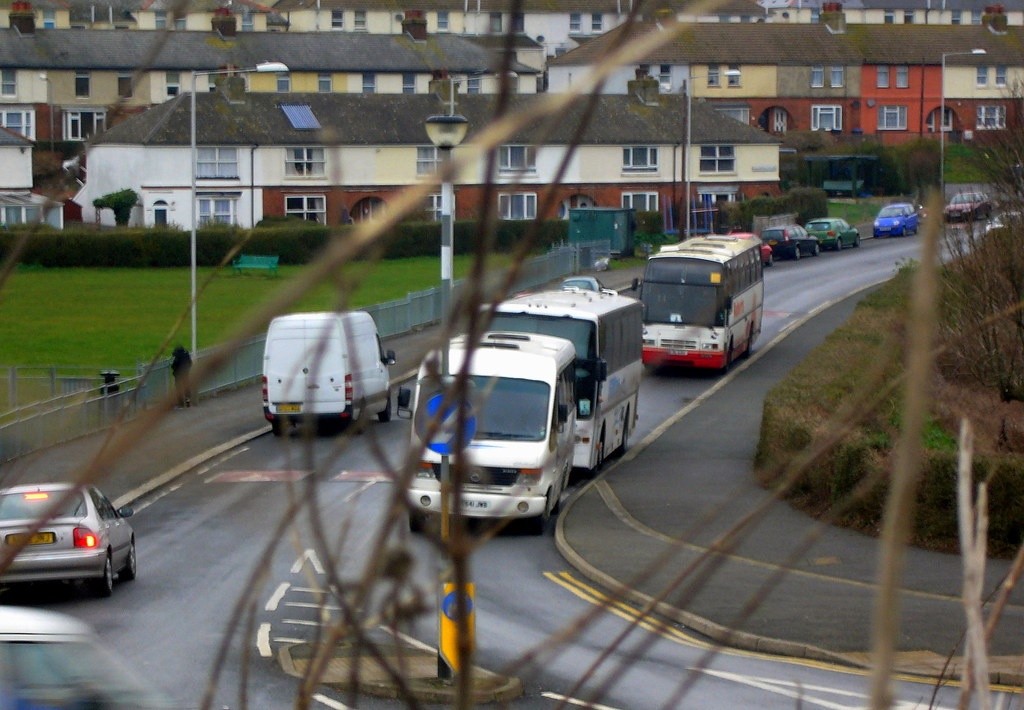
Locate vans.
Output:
[263,311,397,439]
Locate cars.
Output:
[760,224,820,261]
[562,277,604,292]
[0,606,165,710]
[730,233,773,266]
[0,483,136,598]
[873,204,917,239]
[944,192,992,222]
[805,218,861,251]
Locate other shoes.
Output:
[174,406,184,410]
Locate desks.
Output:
[822,180,865,197]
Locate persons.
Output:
[173,341,192,411]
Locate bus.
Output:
[406,333,579,537]
[631,236,766,375]
[480,288,643,476]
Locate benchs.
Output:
[232,254,279,278]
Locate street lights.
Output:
[425,114,467,289]
[191,62,288,362]
[940,49,987,190]
[685,69,743,240]
